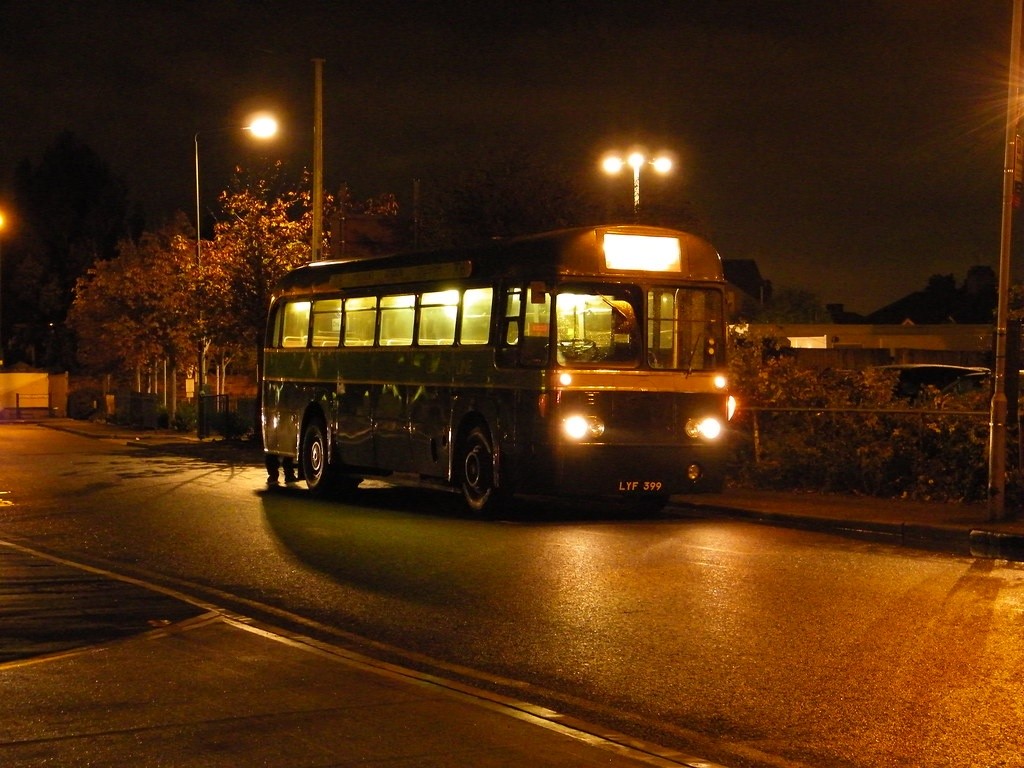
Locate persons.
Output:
[255,381,300,484]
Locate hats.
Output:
[603,297,634,321]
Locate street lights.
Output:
[603,151,676,225]
[195,118,278,438]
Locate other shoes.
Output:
[285,473,299,483]
[267,473,279,483]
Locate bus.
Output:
[257,223,742,520]
